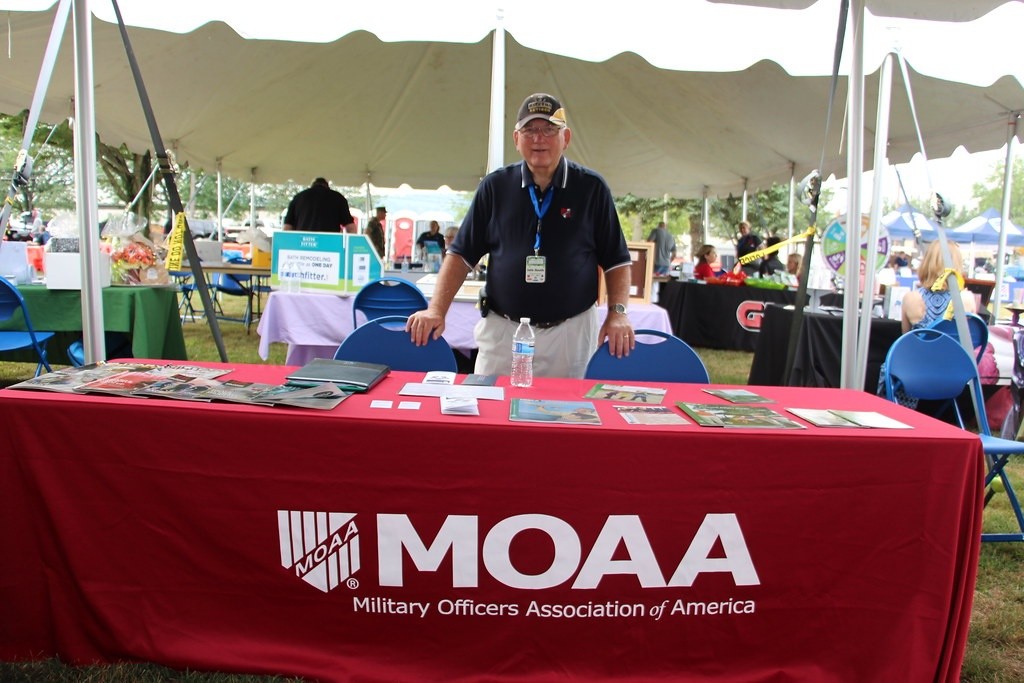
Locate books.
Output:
[509,397,602,425]
[5,359,354,411]
[675,388,914,429]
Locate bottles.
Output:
[401,254,409,271]
[511,318,535,387]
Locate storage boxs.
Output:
[44,253,111,290]
[194,240,222,263]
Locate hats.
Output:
[376,207,387,213]
[694,244,715,258]
[514,93,566,131]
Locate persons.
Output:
[27,209,51,246]
[736,221,803,289]
[415,221,480,273]
[647,221,677,275]
[283,177,357,234]
[894,251,907,273]
[693,245,717,281]
[365,206,388,260]
[405,93,634,379]
[877,237,976,411]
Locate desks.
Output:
[0,358,986,683]
[257,289,672,366]
[748,302,1024,428]
[0,284,188,366]
[181,262,270,323]
[658,281,884,353]
[386,270,476,280]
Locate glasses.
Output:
[519,126,565,138]
[443,236,454,240]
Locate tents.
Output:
[0,1,1024,390]
[879,203,1024,279]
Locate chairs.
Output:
[353,277,429,330]
[0,276,55,378]
[893,312,988,431]
[885,329,1024,541]
[584,330,710,384]
[333,315,458,374]
[168,250,273,335]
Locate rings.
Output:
[624,335,629,338]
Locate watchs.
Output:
[609,304,627,315]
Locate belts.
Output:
[491,309,565,329]
